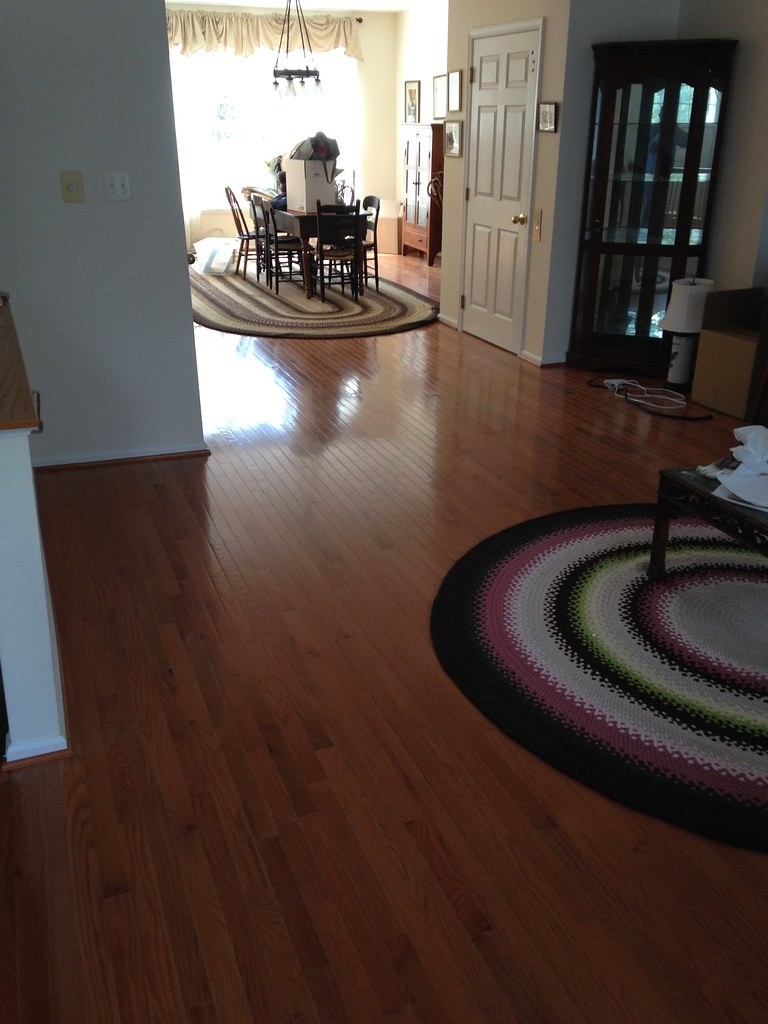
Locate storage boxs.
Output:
[370,198,403,255]
[284,159,335,214]
[689,285,768,421]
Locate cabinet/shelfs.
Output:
[400,122,444,266]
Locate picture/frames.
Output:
[433,74,445,119]
[405,80,420,123]
[447,70,462,112]
[443,120,462,157]
[537,102,557,133]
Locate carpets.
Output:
[188,237,438,339]
[430,502,768,855]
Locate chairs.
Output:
[250,193,303,286]
[225,186,283,280]
[314,186,354,284]
[310,199,361,304]
[261,199,317,296]
[328,196,380,294]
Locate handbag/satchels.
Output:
[289,131,341,184]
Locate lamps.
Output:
[660,276,715,394]
[271,0,322,100]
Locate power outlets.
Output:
[103,173,131,201]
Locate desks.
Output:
[250,202,373,299]
[647,467,768,580]
